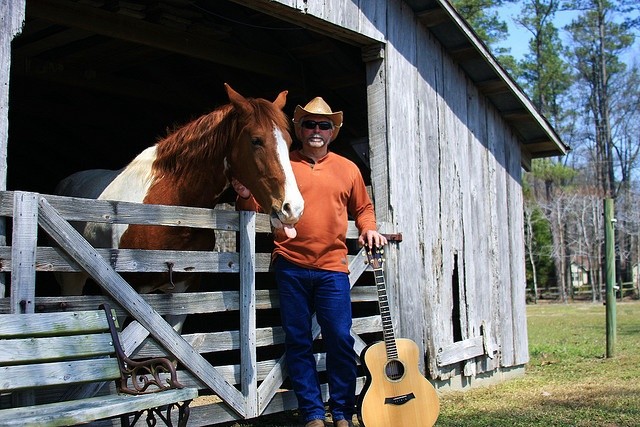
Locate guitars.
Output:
[356,237,440,427]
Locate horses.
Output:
[52,82,305,336]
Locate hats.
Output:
[292,96,344,143]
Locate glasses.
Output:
[301,119,333,131]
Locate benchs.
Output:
[0,303,198,427]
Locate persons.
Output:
[232,95,388,427]
